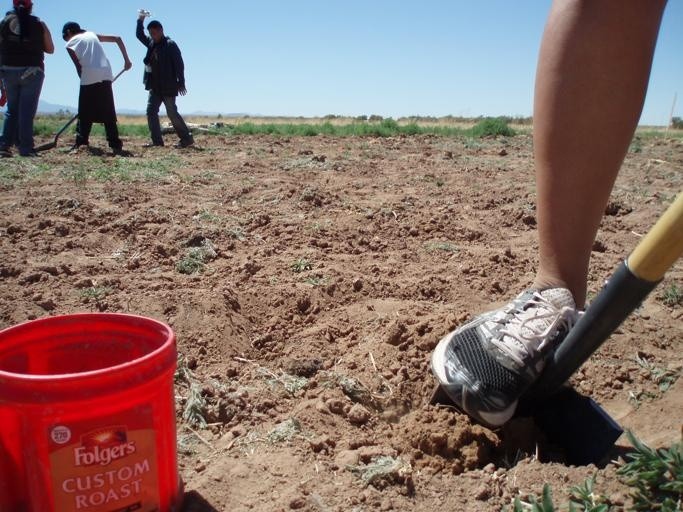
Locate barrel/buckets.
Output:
[1,313,186,512]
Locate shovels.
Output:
[33,63,132,152]
[428,191,683,471]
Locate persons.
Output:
[136,8,195,148]
[62,22,132,155]
[0,1,54,157]
[431,0,683,428]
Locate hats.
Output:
[13,0,33,9]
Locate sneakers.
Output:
[431,285,588,431]
[0,145,13,156]
[175,135,194,147]
[20,149,42,160]
